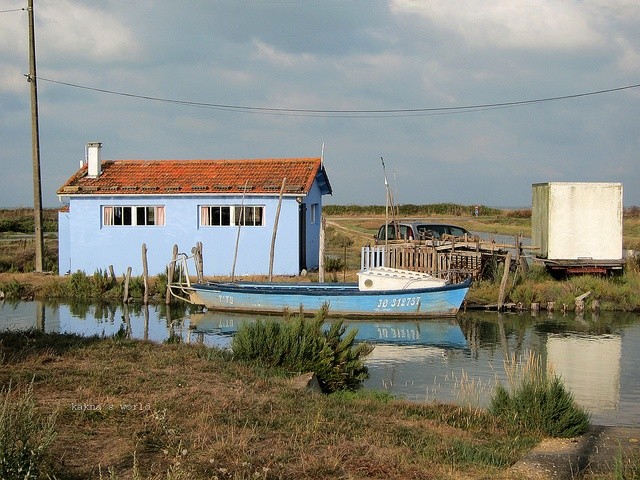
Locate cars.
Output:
[372,221,480,245]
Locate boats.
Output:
[166,250,472,320]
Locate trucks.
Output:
[530,180,626,282]
